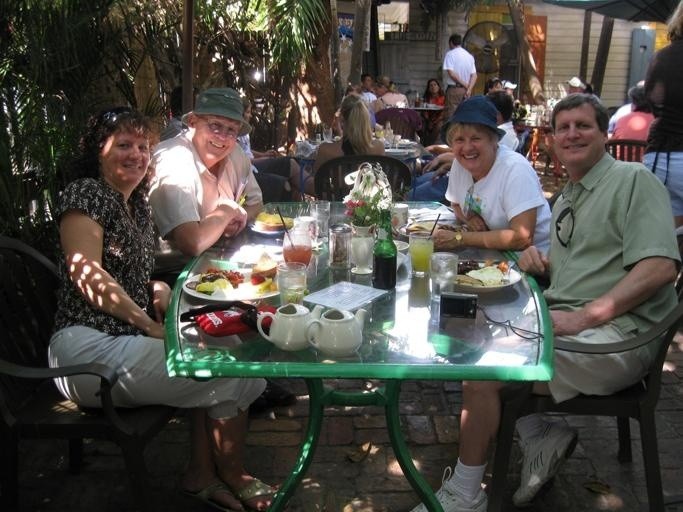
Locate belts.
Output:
[448,84,463,88]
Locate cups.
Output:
[427,296,456,344]
[322,128,332,141]
[409,231,434,279]
[276,199,330,306]
[391,204,409,226]
[315,134,321,142]
[430,251,458,303]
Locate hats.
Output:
[182,88,253,137]
[566,77,587,90]
[501,80,518,90]
[440,95,507,146]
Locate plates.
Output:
[182,269,280,302]
[454,263,522,293]
[250,226,285,238]
[384,149,417,155]
[393,240,409,251]
[397,224,433,239]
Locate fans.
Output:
[461,21,514,94]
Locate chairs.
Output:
[314,155,412,203]
[0,235,177,511]
[374,108,422,141]
[515,127,533,158]
[604,138,648,162]
[487,226,683,512]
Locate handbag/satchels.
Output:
[181,301,277,336]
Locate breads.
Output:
[256,212,293,230]
[252,254,277,285]
[457,265,503,286]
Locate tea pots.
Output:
[257,303,324,352]
[303,309,368,357]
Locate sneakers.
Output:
[410,466,489,512]
[250,378,297,408]
[511,422,578,507]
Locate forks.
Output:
[502,261,516,282]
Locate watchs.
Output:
[455,230,463,248]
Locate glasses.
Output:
[90,109,131,135]
[196,114,239,136]
[555,207,574,248]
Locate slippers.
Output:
[177,481,234,512]
[234,477,291,511]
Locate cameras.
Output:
[439,292,480,321]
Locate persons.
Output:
[160,34,659,204]
[48,107,282,512]
[431,94,552,269]
[643,0,683,250]
[145,87,298,415]
[412,93,683,512]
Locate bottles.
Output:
[370,290,396,362]
[327,223,352,271]
[415,92,420,108]
[408,279,431,311]
[373,209,397,289]
[384,121,393,141]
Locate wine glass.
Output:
[348,237,373,275]
